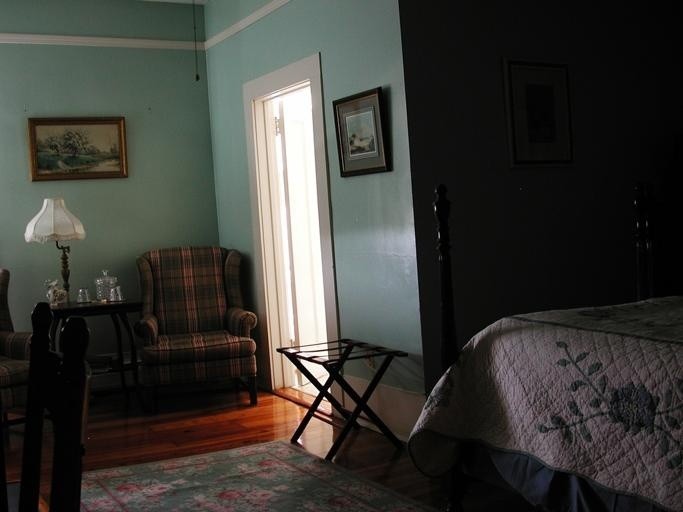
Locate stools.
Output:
[276,338,405,461]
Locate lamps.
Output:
[22,195,85,303]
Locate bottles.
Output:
[109,277,123,301]
[95,270,120,300]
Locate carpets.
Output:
[41,437,445,511]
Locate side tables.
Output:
[49,299,144,408]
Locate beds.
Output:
[405,181,683,512]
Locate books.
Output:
[85,355,111,375]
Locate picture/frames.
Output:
[332,86,389,178]
[501,54,577,170]
[26,117,129,182]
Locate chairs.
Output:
[0,268,35,408]
[1,299,90,511]
[136,243,258,410]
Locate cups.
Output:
[78,287,90,303]
